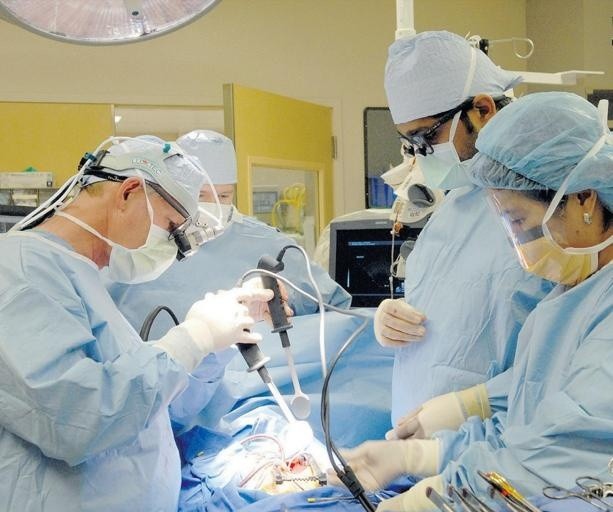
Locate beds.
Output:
[174,306,541,512]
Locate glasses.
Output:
[142,179,192,241]
[396,114,453,159]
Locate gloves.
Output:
[374,297,426,349]
[327,441,442,512]
[385,381,492,440]
[182,279,294,363]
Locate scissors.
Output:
[543,476,613,512]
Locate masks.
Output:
[194,203,233,231]
[413,114,477,191]
[511,191,613,287]
[98,181,181,286]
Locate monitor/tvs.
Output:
[329,208,423,309]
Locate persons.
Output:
[95,130,353,464]
[323,93,613,511]
[0,133,294,512]
[371,31,555,442]
